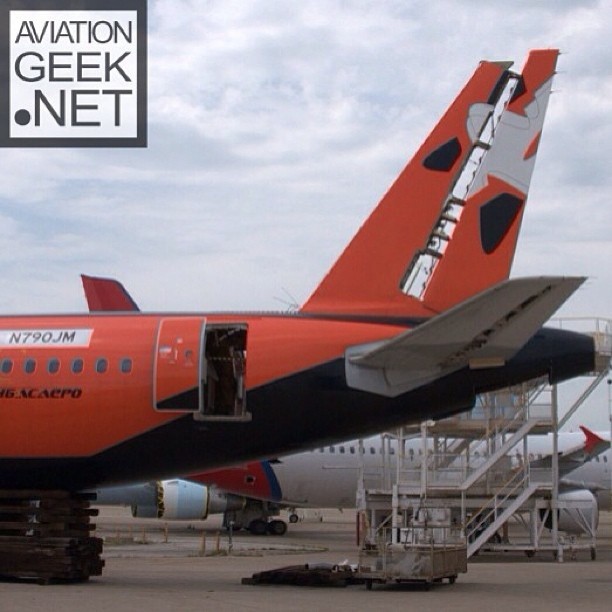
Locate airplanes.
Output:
[80,387,612,556]
[0,45,612,497]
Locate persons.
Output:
[212,331,237,415]
[229,329,244,413]
